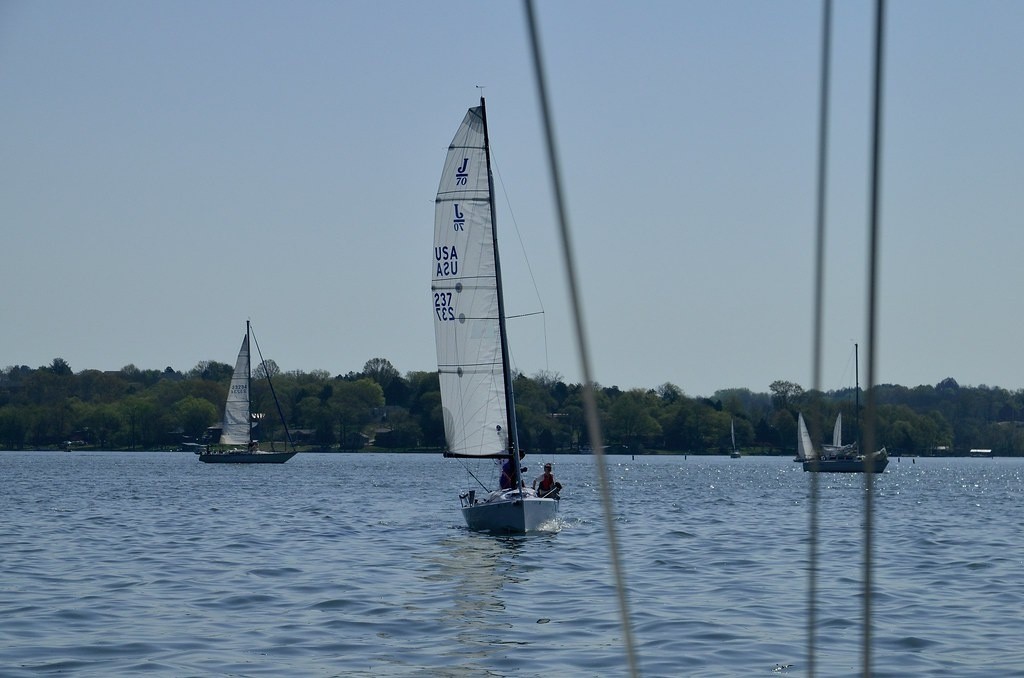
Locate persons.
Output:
[499,449,527,489]
[533,463,561,500]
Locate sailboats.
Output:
[199,319,300,464]
[730,417,742,460]
[788,345,890,475]
[428,94,559,537]
